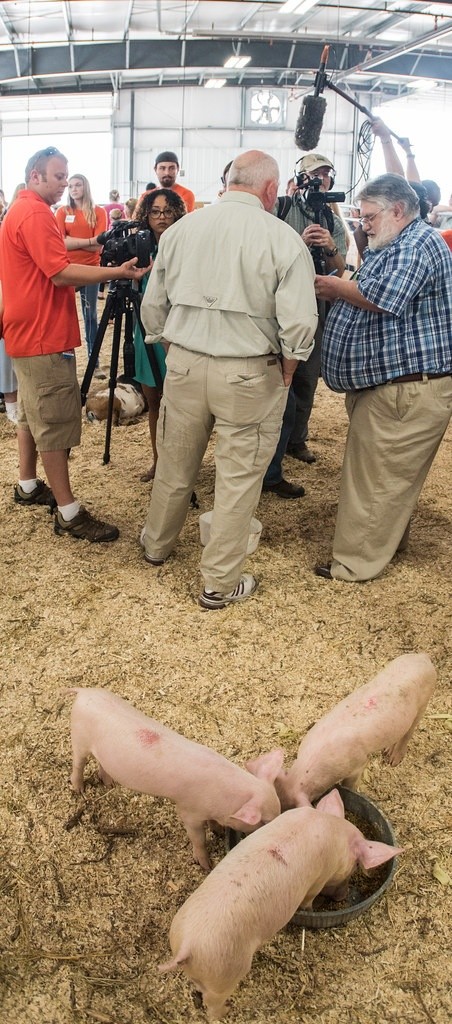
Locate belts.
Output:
[392,372,452,384]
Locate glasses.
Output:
[33,146,59,169]
[309,171,331,178]
[148,209,175,219]
[359,206,390,225]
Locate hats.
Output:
[296,154,335,175]
[156,151,178,163]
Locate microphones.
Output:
[298,178,323,189]
[294,46,329,152]
[95,230,114,245]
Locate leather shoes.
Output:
[261,479,305,498]
[286,443,317,463]
[316,566,333,577]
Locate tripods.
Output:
[68,262,165,464]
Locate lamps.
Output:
[224,55,252,69]
[204,79,226,89]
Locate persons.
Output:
[315,172,452,580]
[0,146,452,545]
[122,150,319,609]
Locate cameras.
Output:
[306,190,345,211]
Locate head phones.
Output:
[294,156,336,190]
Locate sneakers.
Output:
[200,572,257,609]
[14,478,55,505]
[140,526,166,566]
[54,504,119,542]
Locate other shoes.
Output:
[6,410,18,424]
[98,296,104,299]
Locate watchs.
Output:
[325,245,338,257]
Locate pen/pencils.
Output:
[330,269,339,275]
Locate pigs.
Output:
[156,788,405,1019]
[61,685,283,870]
[86,376,145,426]
[273,652,437,809]
